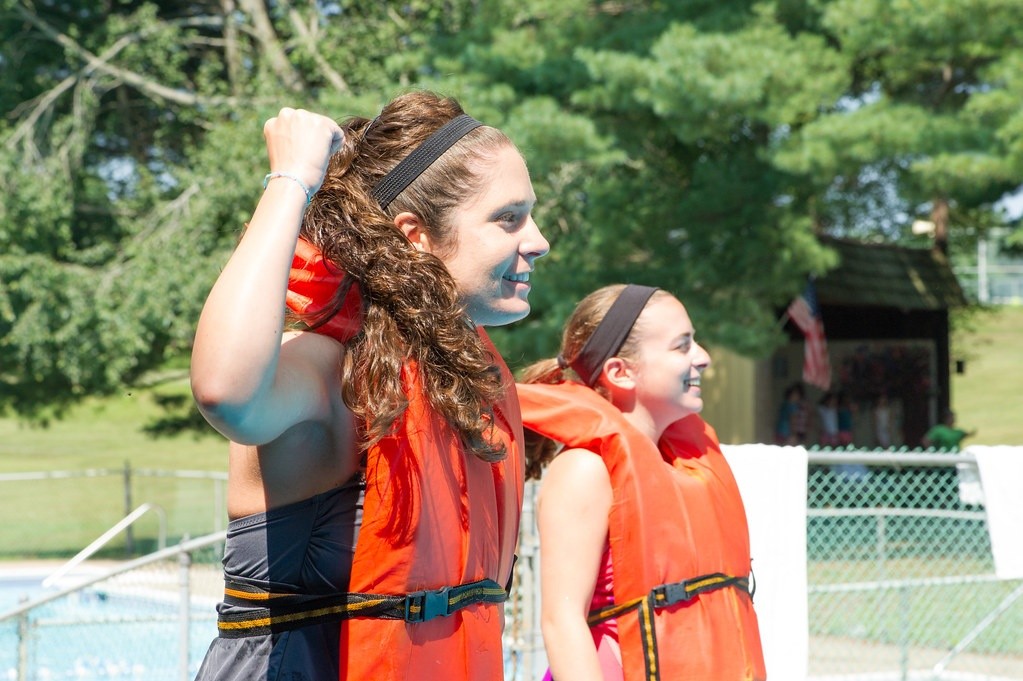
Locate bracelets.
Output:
[263,171,312,208]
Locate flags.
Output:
[784,277,834,389]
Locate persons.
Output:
[188,91,551,681]
[519,283,768,681]
[781,346,978,457]
